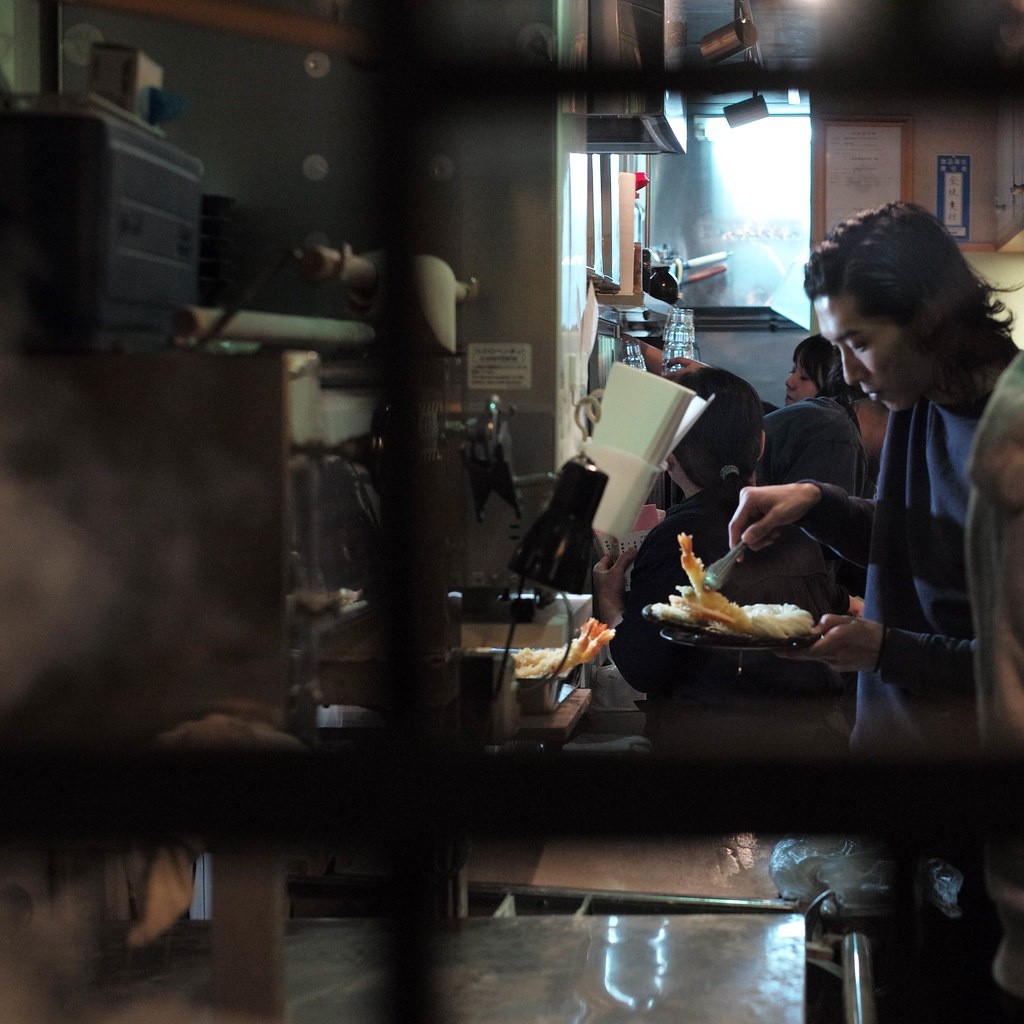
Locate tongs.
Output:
[702,536,754,592]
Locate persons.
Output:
[615,333,842,417]
[964,351,1024,1024]
[726,200,1024,759]
[752,358,898,624]
[592,366,836,726]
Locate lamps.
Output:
[723,44,770,127]
[699,0,758,65]
[448,394,610,593]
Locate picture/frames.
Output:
[811,113,916,254]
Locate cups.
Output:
[662,342,694,377]
[662,307,695,342]
[621,340,648,372]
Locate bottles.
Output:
[649,265,679,304]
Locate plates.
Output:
[643,604,824,653]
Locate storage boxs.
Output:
[0,91,208,360]
